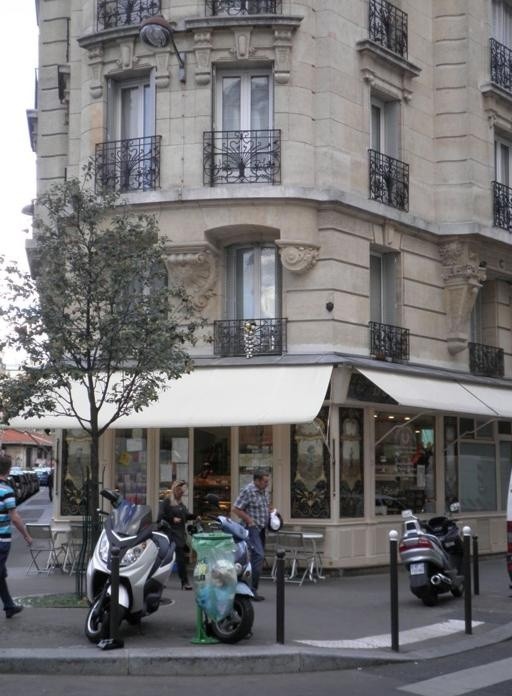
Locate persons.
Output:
[157,479,202,591]
[0,456,36,620]
[231,468,272,602]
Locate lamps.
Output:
[138,13,186,84]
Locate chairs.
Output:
[259,523,327,587]
[23,520,92,576]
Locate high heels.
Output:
[180,581,193,590]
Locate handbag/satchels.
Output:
[158,518,176,536]
[268,511,283,532]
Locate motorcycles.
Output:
[399,502,466,607]
[187,493,264,644]
[84,486,177,642]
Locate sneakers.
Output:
[251,594,265,601]
[5,604,23,618]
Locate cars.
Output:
[7,466,54,503]
